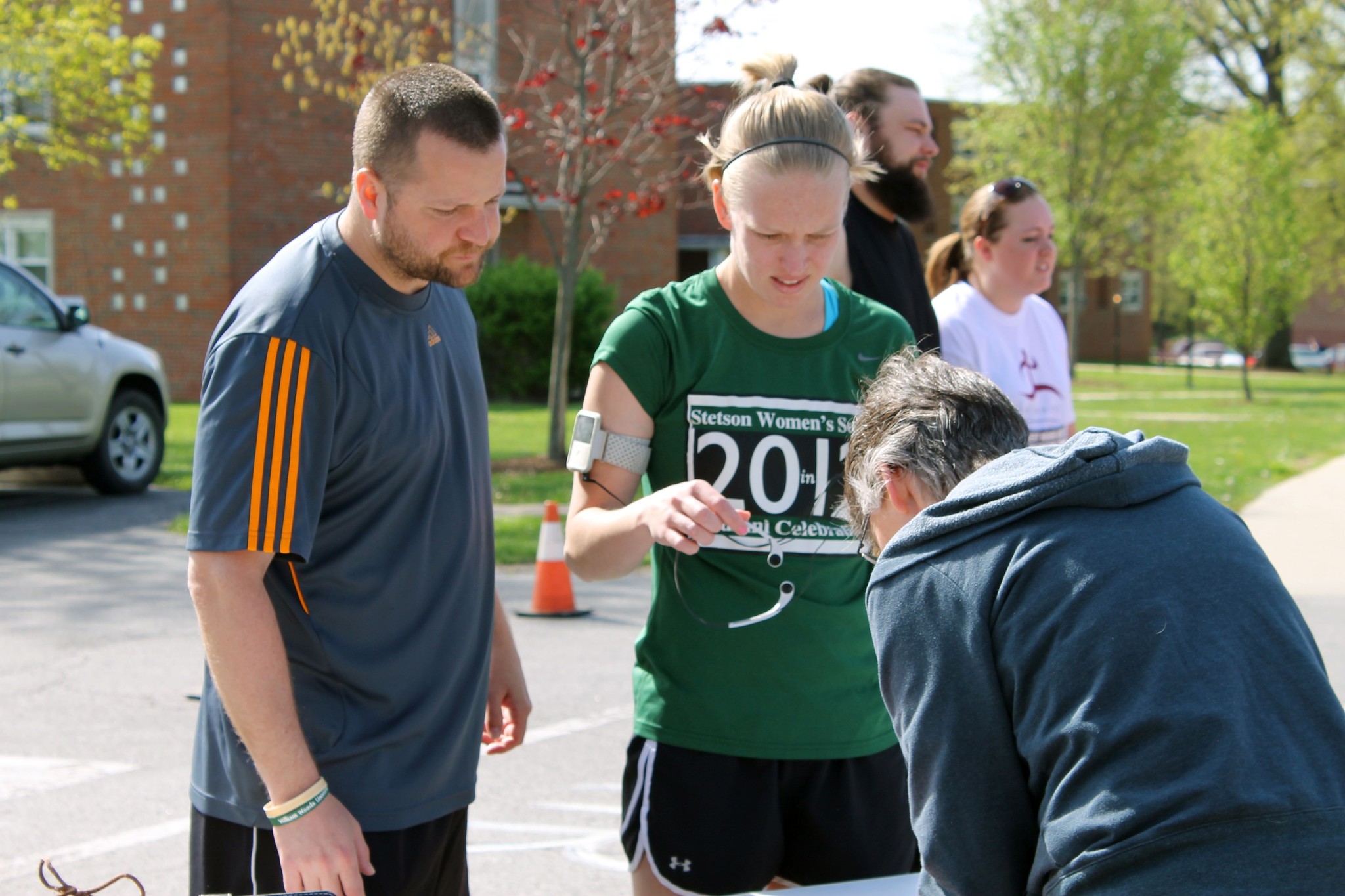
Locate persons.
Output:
[843,345,1345,896]
[564,55,920,896]
[186,63,533,896]
[804,68,942,357]
[924,179,1076,446]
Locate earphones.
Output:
[742,522,783,568]
[727,579,793,629]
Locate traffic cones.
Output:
[515,500,597,618]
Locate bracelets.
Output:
[268,786,330,827]
[262,776,328,818]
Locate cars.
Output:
[1177,341,1243,368]
[1288,344,1345,372]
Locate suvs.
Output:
[1,253,172,496]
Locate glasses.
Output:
[980,179,1036,238]
[857,510,877,565]
